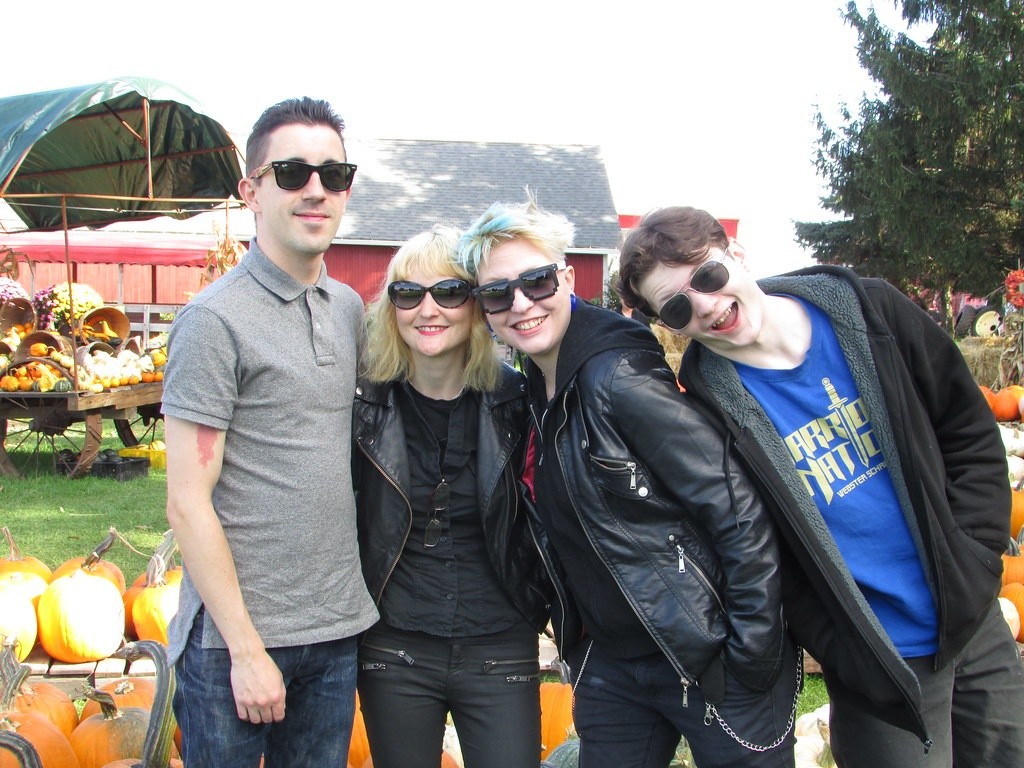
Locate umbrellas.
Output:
[3,232,237,305]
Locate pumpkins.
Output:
[0,525,183,768]
[346,689,374,768]
[540,663,576,761]
[979,384,1024,646]
[0,316,167,392]
[58,439,165,480]
[441,749,460,768]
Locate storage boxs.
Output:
[118,447,166,469]
[56,457,149,480]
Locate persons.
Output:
[350,223,557,767]
[614,206,1023,768]
[160,96,367,768]
[457,198,811,768]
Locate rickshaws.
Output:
[0,353,160,483]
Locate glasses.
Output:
[645,245,730,331]
[248,160,357,192]
[423,476,451,549]
[388,278,476,310]
[472,262,566,315]
